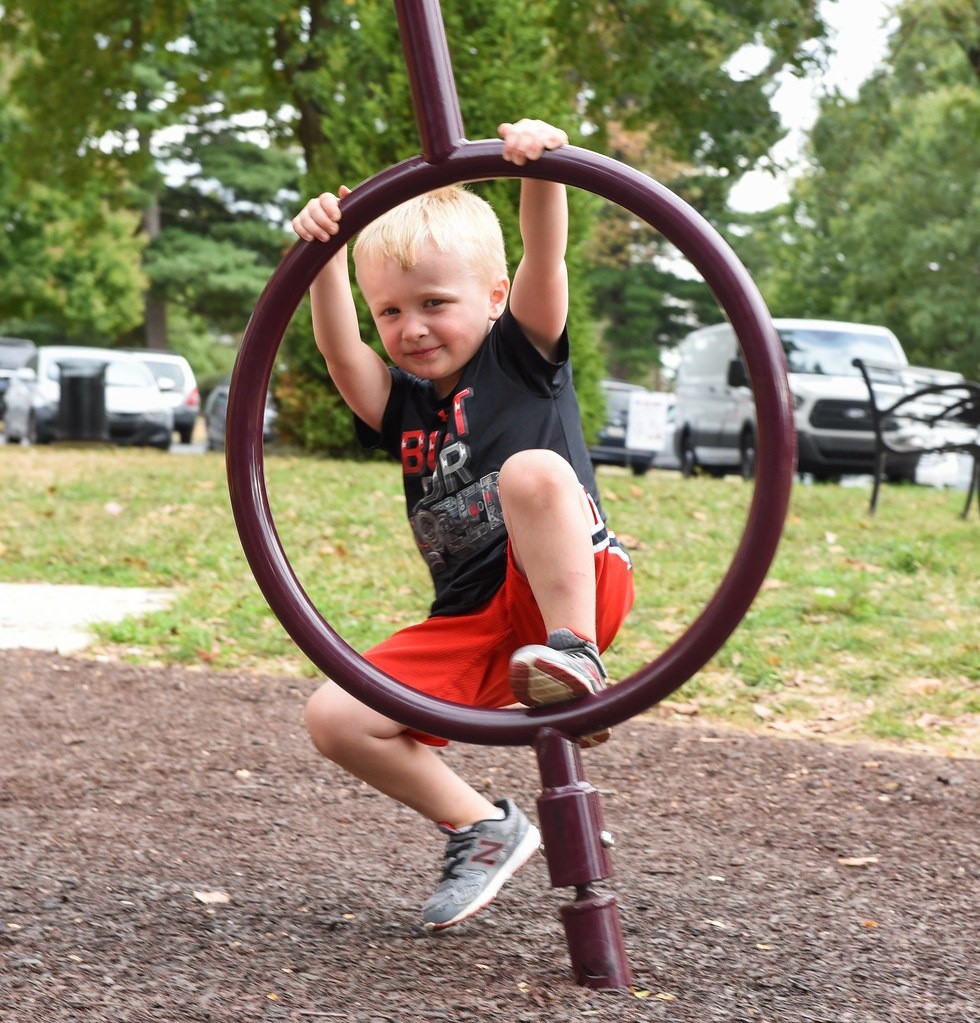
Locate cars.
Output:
[2,346,174,450]
[649,391,684,470]
[0,337,37,414]
[204,385,279,451]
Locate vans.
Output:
[132,352,201,442]
[590,381,656,475]
[673,316,927,484]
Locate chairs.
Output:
[851,357,979,520]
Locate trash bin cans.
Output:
[57,359,111,440]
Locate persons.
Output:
[291,118,635,933]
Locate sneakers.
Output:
[422,798,541,932]
[507,627,611,749]
